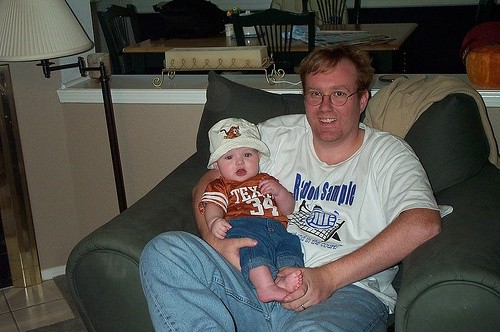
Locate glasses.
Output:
[302,89,360,107]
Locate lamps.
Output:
[0,0,128,213]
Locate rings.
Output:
[301,304,306,310]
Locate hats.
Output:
[206,118,271,169]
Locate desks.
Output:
[121,22,419,74]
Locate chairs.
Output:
[97,0,361,75]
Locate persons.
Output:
[137,43,455,332]
[197,117,306,304]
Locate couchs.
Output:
[64,72,500,332]
